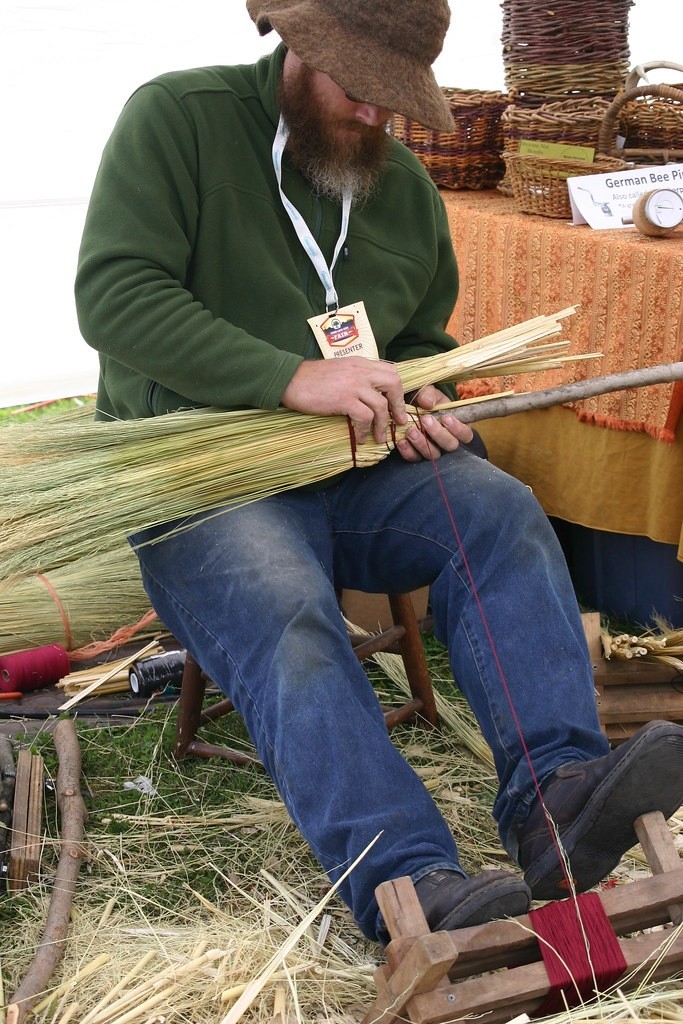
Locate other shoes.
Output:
[412,869,533,932]
[519,719,683,901]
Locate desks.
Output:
[438,188,683,570]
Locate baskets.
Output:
[388,85,509,188]
[498,2,633,106]
[620,62,683,151]
[597,83,683,169]
[501,96,622,158]
[500,149,635,219]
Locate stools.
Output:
[172,588,439,769]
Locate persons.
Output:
[72,0,682,949]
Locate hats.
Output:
[246,1,458,133]
